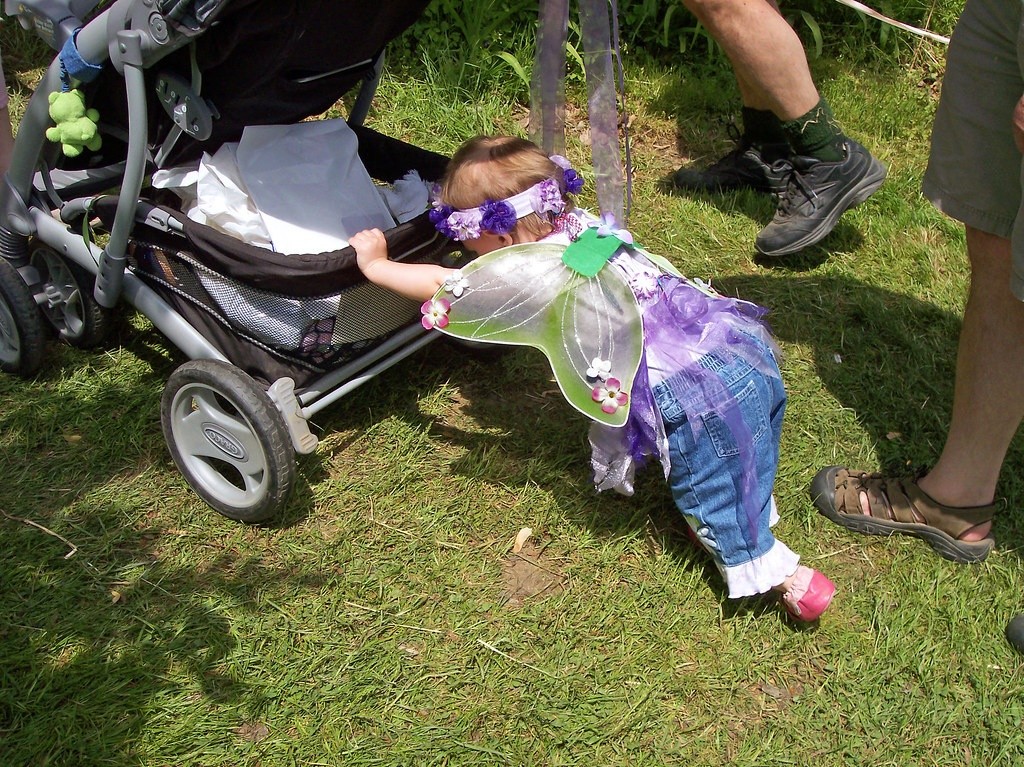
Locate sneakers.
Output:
[753,137,886,257]
[667,123,795,198]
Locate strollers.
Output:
[1,0,528,524]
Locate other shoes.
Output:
[782,566,835,623]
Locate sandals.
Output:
[811,465,1011,564]
[1004,611,1024,656]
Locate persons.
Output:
[812,1,1023,648]
[669,0,885,256]
[1,61,16,183]
[349,137,838,620]
